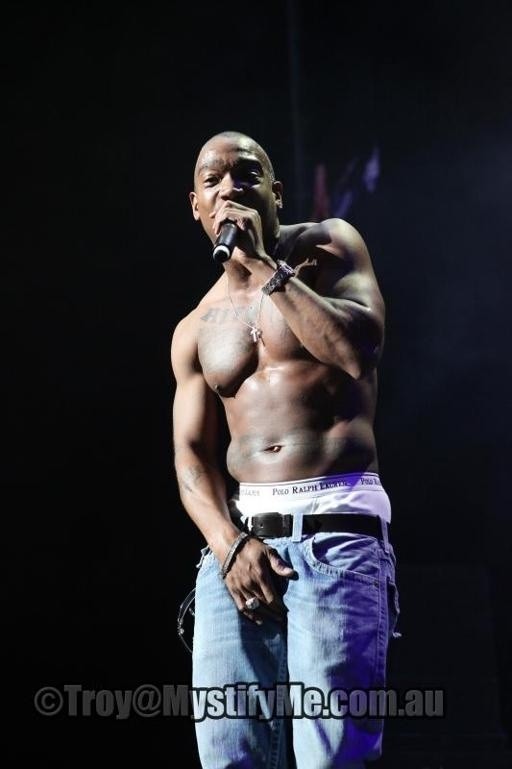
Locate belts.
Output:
[245,514,390,539]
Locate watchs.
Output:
[260,259,296,294]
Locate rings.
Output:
[242,596,260,611]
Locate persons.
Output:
[170,128,400,769]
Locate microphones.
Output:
[211,220,238,262]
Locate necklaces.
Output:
[227,273,263,343]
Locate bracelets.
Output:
[220,532,249,578]
